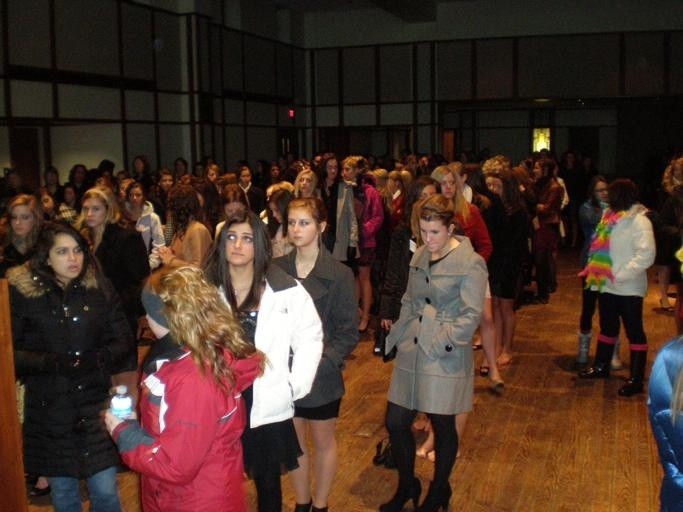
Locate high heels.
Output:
[383,448,398,468]
[416,480,450,508]
[359,316,372,337]
[375,472,420,508]
[374,438,388,465]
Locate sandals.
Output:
[659,299,674,313]
[30,478,51,496]
[490,372,504,388]
[479,359,490,377]
[473,340,482,351]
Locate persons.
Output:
[178,174,210,183]
[65,164,88,189]
[453,148,481,159]
[288,155,321,169]
[238,166,265,215]
[206,161,220,182]
[174,158,188,176]
[121,182,166,270]
[342,155,384,331]
[379,177,464,331]
[569,149,597,170]
[133,154,151,182]
[92,167,130,183]
[2,170,31,196]
[149,168,175,223]
[62,183,80,211]
[118,178,137,200]
[380,195,488,505]
[316,159,360,261]
[375,407,468,462]
[472,332,488,376]
[430,166,505,389]
[222,173,238,183]
[1,210,14,241]
[270,196,360,505]
[215,184,251,234]
[194,162,208,176]
[322,151,336,158]
[202,209,324,512]
[253,158,267,181]
[372,169,394,216]
[648,334,683,511]
[295,169,318,198]
[4,195,44,263]
[577,175,626,369]
[530,158,562,303]
[73,190,149,326]
[581,179,655,396]
[34,186,78,222]
[543,160,570,209]
[158,184,213,269]
[42,166,63,201]
[445,161,503,210]
[6,222,137,510]
[390,171,412,215]
[268,189,295,240]
[276,156,291,179]
[266,180,293,203]
[105,266,265,511]
[481,153,535,176]
[532,143,563,157]
[368,154,431,170]
[478,172,524,365]
[261,164,284,187]
[655,158,683,312]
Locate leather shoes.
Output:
[373,332,386,356]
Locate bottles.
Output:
[110,385,132,419]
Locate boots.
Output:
[618,343,650,395]
[577,335,616,378]
[608,339,622,370]
[572,333,592,365]
